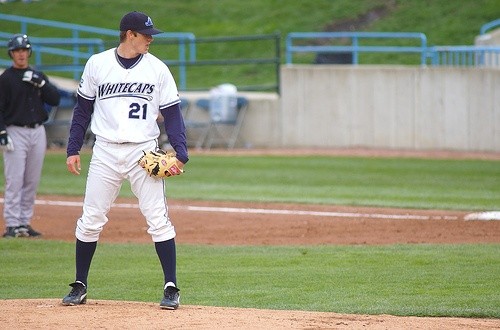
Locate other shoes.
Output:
[3,225,40,238]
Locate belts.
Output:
[10,122,42,128]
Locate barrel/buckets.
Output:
[209,84,237,122]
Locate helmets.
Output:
[8,34,33,58]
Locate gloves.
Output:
[0,130,8,145]
[22,71,45,88]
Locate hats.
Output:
[120,11,163,35]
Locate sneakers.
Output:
[160,286,180,309]
[61,282,87,305]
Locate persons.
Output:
[0,33,60,239]
[62,10,190,310]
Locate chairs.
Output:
[178,97,251,151]
[44,90,76,147]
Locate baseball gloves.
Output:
[137,148,186,179]
